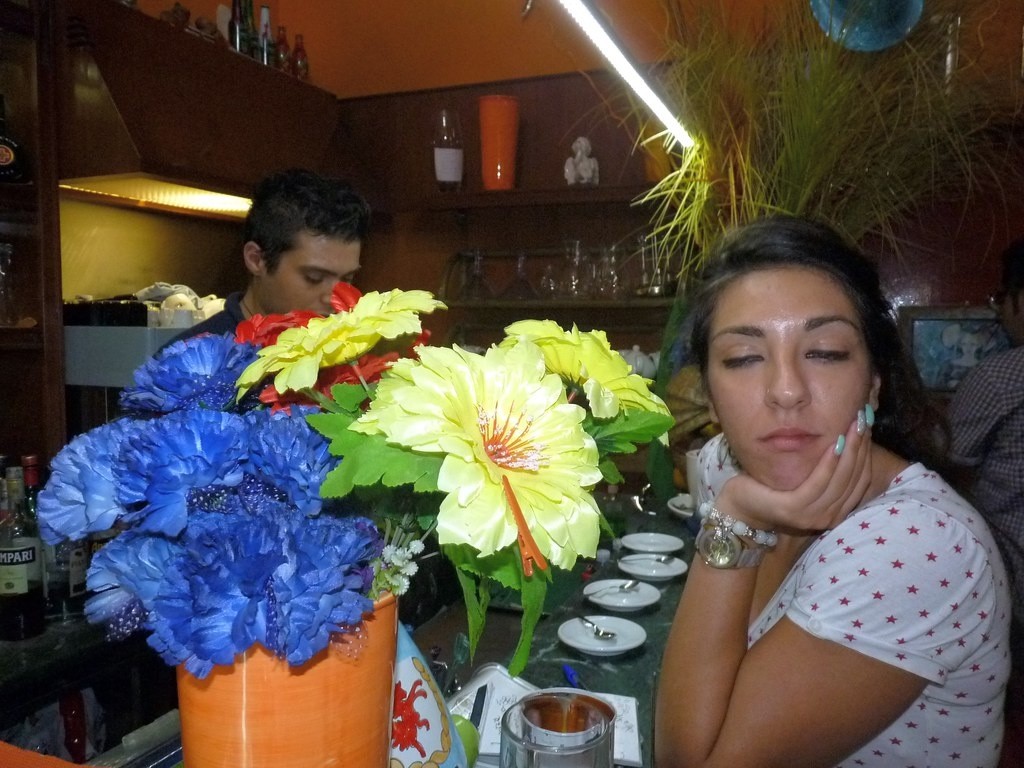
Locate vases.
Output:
[177,592,399,768]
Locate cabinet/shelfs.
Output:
[0,0,69,660]
[436,251,692,483]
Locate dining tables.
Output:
[413,503,709,768]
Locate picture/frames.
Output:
[896,302,1010,408]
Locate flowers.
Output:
[30,288,677,678]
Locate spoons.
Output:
[582,578,640,599]
[572,611,615,639]
[608,554,674,564]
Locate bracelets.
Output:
[700,503,778,547]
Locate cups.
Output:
[499,687,618,767]
[686,449,700,491]
[477,93,522,190]
[147,307,205,327]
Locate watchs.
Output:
[695,520,766,569]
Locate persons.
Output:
[141,166,374,420]
[937,238,1024,715]
[654,215,1013,768]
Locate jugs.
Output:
[616,342,656,379]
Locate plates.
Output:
[617,554,688,582]
[557,615,648,656]
[666,495,694,521]
[620,533,685,554]
[583,580,661,613]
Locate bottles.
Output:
[600,485,627,541]
[431,107,464,197]
[0,450,126,642]
[229,0,309,84]
[436,235,685,297]
